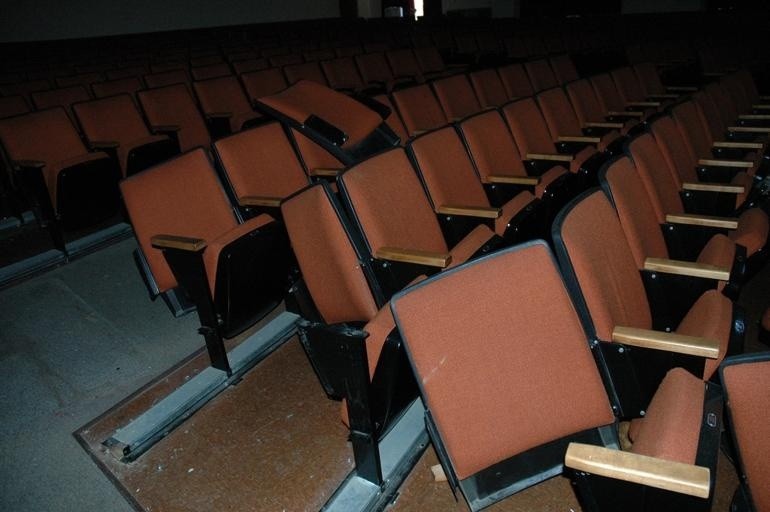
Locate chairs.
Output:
[383,238,729,510]
[716,345,769,512]
[269,175,429,490]
[0,11,769,370]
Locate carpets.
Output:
[73,291,745,511]
